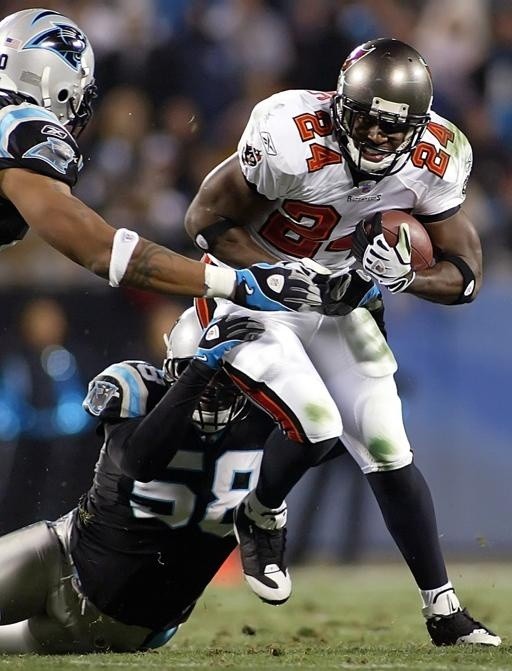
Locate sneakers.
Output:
[421,602,502,648]
[232,493,292,603]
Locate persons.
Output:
[183,36,505,652]
[0,0,478,537]
[0,297,348,653]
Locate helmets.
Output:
[161,305,257,441]
[1,8,99,140]
[330,39,434,181]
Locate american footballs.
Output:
[365,210,433,271]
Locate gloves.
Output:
[195,314,266,364]
[352,211,416,295]
[233,262,381,315]
